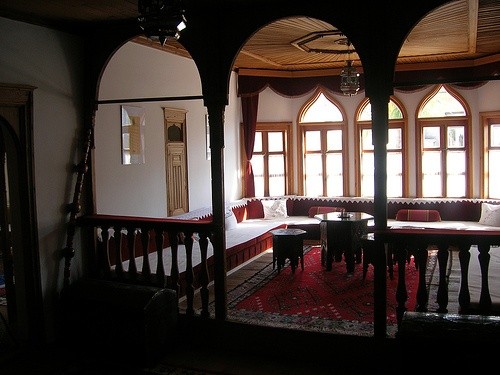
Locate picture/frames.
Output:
[206,114,211,160]
[122,105,145,165]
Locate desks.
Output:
[313,211,374,276]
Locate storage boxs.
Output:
[397,311,500,375]
[66,278,178,368]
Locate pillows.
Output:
[396,209,441,221]
[224,205,238,230]
[308,207,345,218]
[261,199,288,219]
[478,203,500,227]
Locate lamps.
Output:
[137,0,188,47]
[339,37,360,99]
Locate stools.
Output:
[270,228,307,273]
[360,232,394,280]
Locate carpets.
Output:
[195,244,453,338]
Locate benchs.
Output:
[97,195,500,317]
